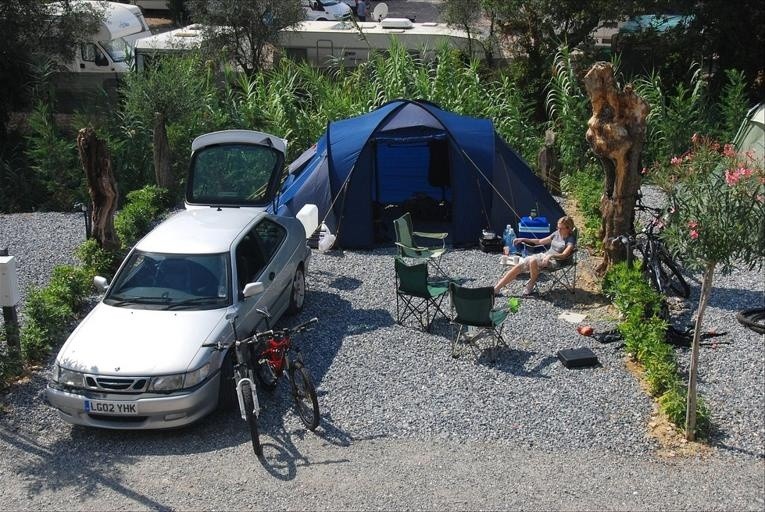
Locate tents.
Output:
[266,97,565,251]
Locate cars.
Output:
[45,128,312,431]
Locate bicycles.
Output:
[201,306,273,458]
[632,203,691,319]
[249,304,321,433]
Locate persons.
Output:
[492,216,575,297]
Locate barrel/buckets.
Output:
[320,221,331,243]
[503,224,520,254]
[296,203,319,240]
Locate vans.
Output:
[34,1,154,109]
[297,0,370,22]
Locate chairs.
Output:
[392,212,449,333]
[449,283,509,366]
[521,226,579,298]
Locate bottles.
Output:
[318,229,336,254]
